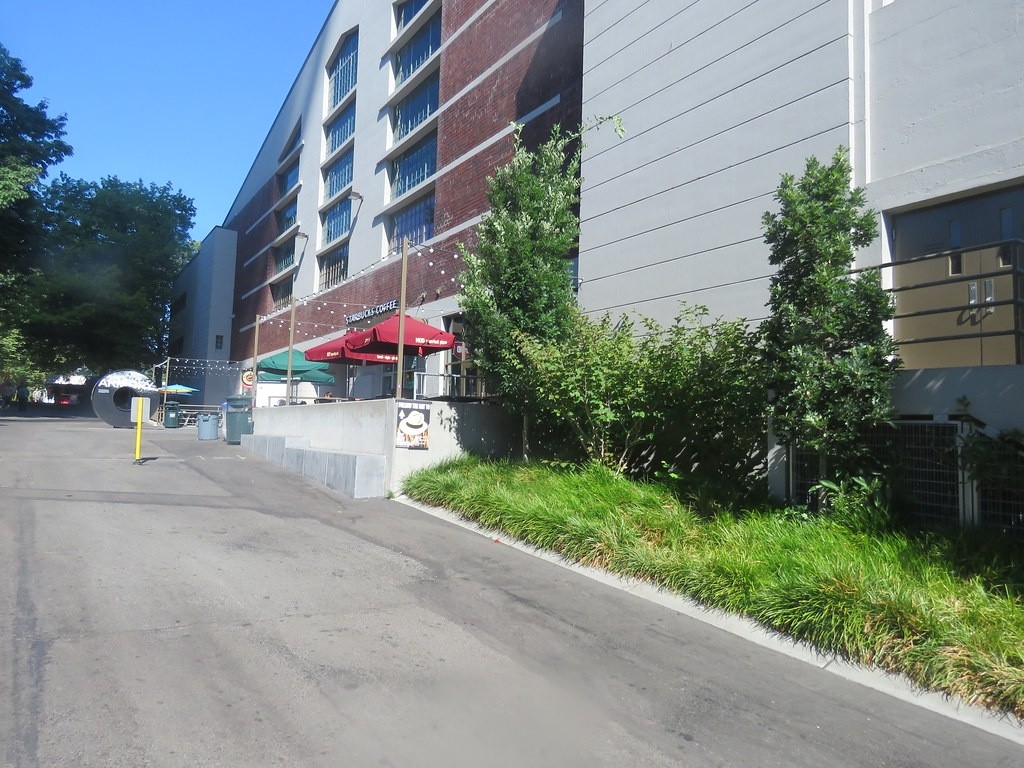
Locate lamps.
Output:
[296,232,309,241]
[347,191,364,201]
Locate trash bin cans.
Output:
[222,402,242,440]
[197,412,219,440]
[226,394,253,442]
[164,401,179,428]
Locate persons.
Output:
[0,376,15,409]
[325,392,333,403]
[15,382,29,414]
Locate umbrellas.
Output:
[306,329,399,400]
[257,346,330,404]
[341,313,456,388]
[157,384,200,405]
[257,367,334,404]
